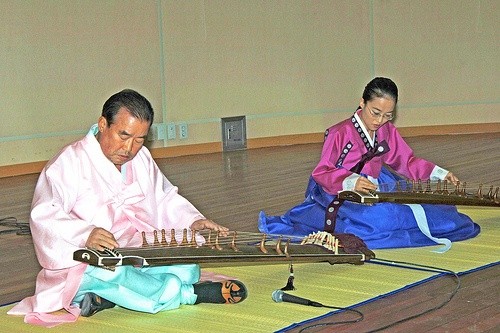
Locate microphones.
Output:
[271,290,323,307]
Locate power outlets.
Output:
[147,124,187,141]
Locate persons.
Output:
[6,89,248,316]
[256,77,481,249]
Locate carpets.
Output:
[0,205,500,333]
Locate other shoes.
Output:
[81,291,117,317]
[193,280,247,304]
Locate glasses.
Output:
[365,105,394,120]
